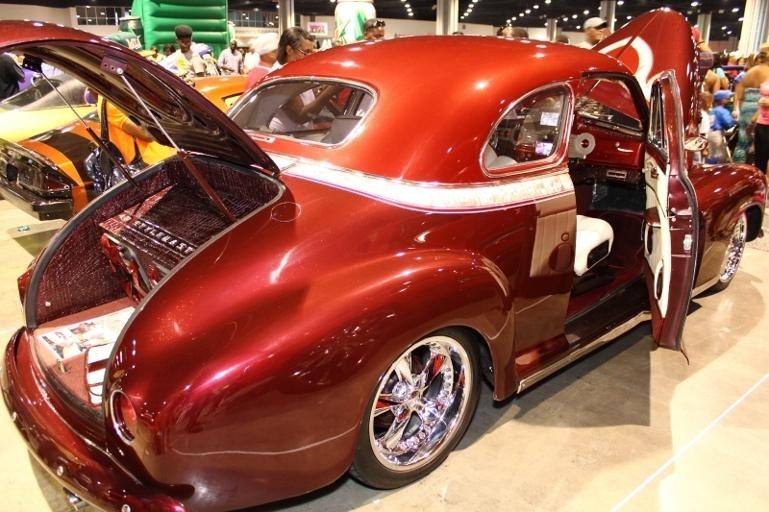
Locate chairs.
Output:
[483,142,615,275]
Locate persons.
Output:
[0,50,64,102]
[574,16,612,50]
[693,39,769,175]
[362,19,386,41]
[94,94,154,196]
[146,25,346,132]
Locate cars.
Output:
[0,6,769,512]
[0,72,248,222]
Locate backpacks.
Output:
[84,98,149,195]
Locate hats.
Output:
[583,17,607,29]
[254,32,280,56]
[713,90,735,101]
